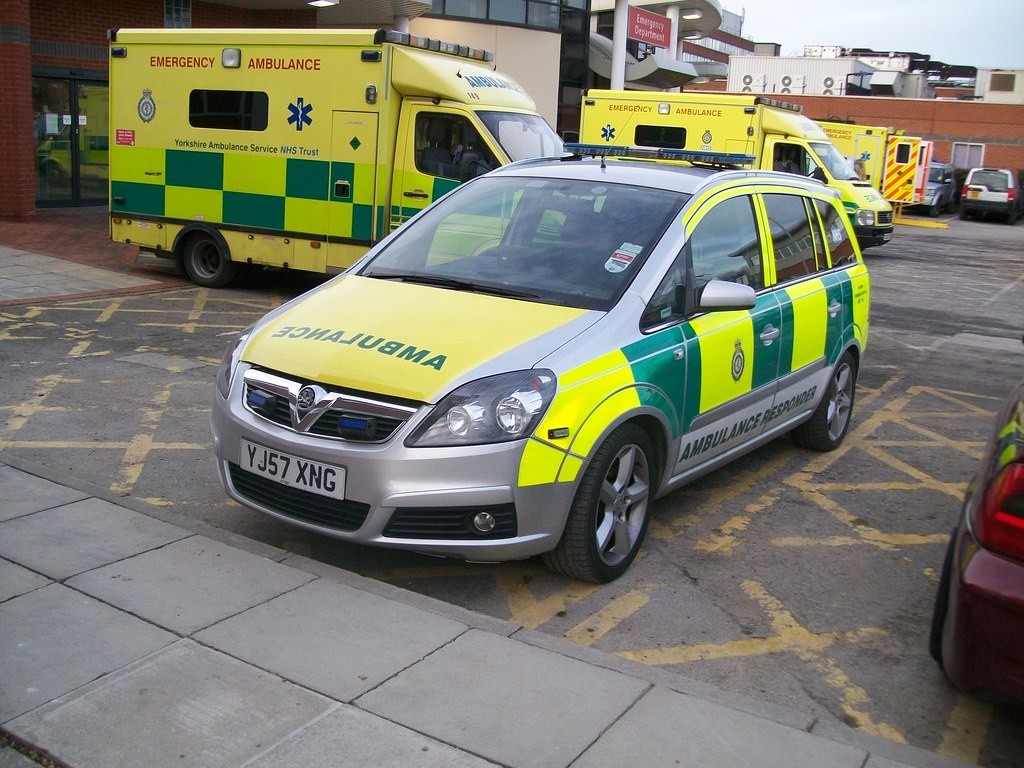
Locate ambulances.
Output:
[578,86,896,251]
[211,139,872,586]
[36,85,108,194]
[106,25,638,289]
[806,117,934,219]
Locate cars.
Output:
[926,378,1024,711]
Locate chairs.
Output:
[556,195,670,289]
[419,119,457,180]
[773,161,800,175]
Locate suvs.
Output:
[959,167,1022,225]
[903,160,957,217]
